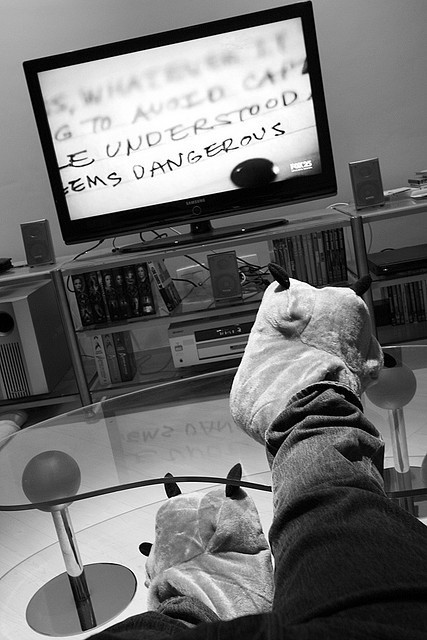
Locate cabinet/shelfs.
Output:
[0,191,427,423]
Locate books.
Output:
[273,229,347,290]
[381,280,426,323]
[92,330,137,383]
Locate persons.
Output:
[90,262,426,640]
[71,266,155,321]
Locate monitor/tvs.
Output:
[21,0,340,252]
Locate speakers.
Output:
[22,220,57,267]
[0,280,75,402]
[206,253,244,305]
[350,157,385,209]
